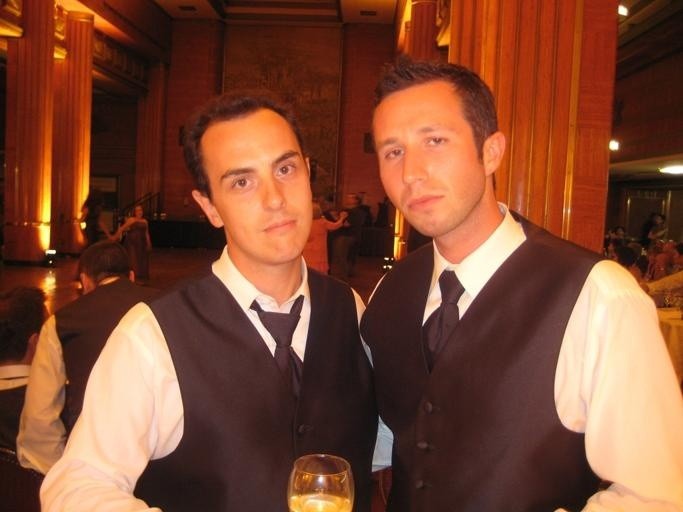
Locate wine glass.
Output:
[663,294,671,308]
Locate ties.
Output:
[421,271,464,371]
[250,296,303,397]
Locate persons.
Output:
[1,287,52,455]
[98,205,155,283]
[604,210,683,294]
[35,83,392,512]
[16,242,175,476]
[334,62,683,512]
[303,194,365,275]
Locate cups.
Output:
[286,453,356,512]
